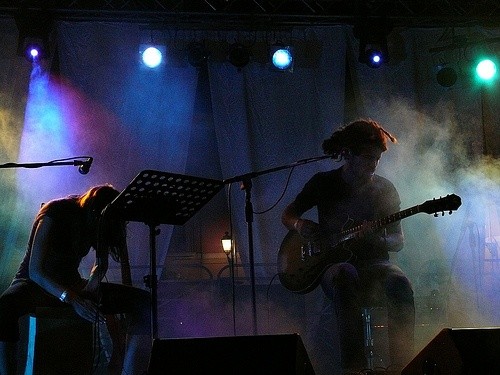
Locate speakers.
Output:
[400,325,500,375]
[144,332,317,375]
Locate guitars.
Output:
[276,193,462,294]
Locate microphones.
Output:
[78,157,93,174]
[330,151,342,162]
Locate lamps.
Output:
[352,20,393,68]
[227,30,257,66]
[172,29,210,65]
[431,53,457,87]
[140,27,162,69]
[268,29,294,70]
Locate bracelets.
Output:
[59,289,70,302]
[294,219,300,229]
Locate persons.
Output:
[0,184,152,375]
[282,119,415,375]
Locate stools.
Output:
[17,307,94,375]
[338,298,387,375]
